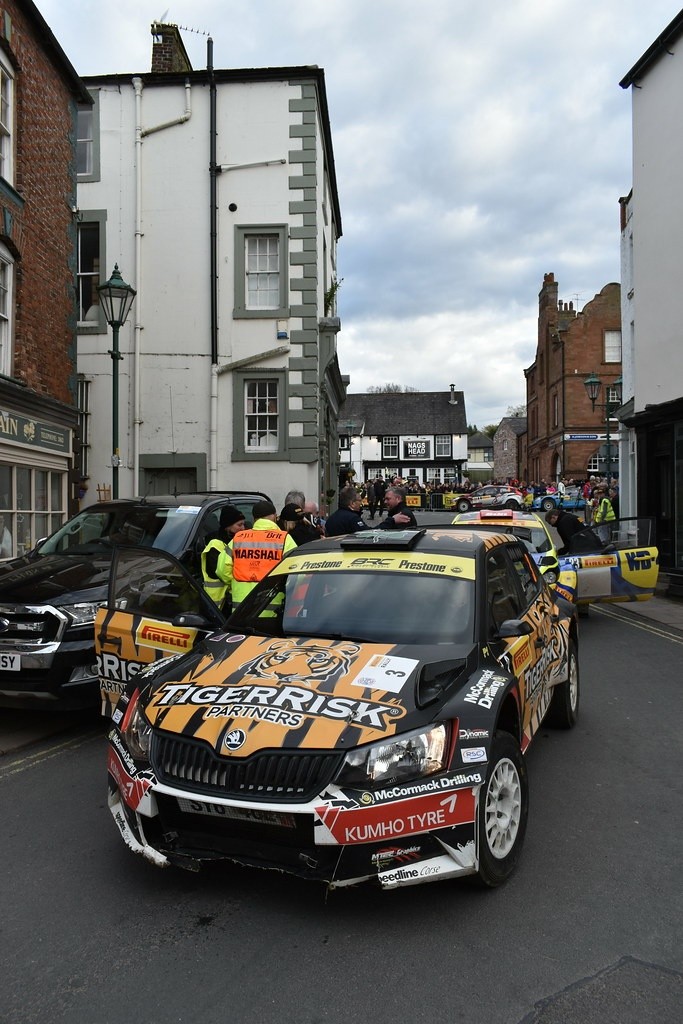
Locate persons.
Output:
[244,521,253,530]
[98,518,130,545]
[344,472,621,544]
[546,509,606,620]
[383,487,416,529]
[324,487,410,538]
[216,502,299,616]
[201,505,245,612]
[274,490,326,546]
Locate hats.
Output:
[280,502,305,521]
[219,505,245,528]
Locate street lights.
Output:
[95,263,138,503]
[585,371,622,498]
[345,424,355,469]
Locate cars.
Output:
[450,510,661,611]
[452,485,525,514]
[99,526,586,894]
[532,490,587,510]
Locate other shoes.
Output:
[367,517,374,520]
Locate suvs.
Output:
[0,492,290,705]
